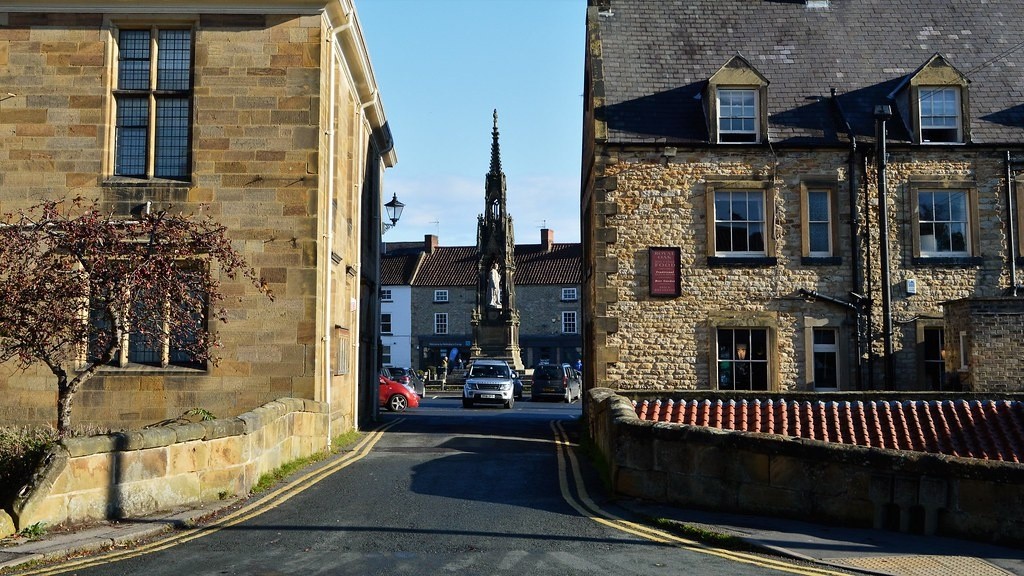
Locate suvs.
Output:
[461,361,516,409]
[389,367,426,399]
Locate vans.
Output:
[530,363,580,403]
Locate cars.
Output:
[496,370,523,397]
[574,370,582,395]
[380,367,394,381]
[379,374,421,413]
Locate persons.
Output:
[574,359,583,376]
[442,356,449,383]
[458,359,464,369]
[488,262,502,307]
